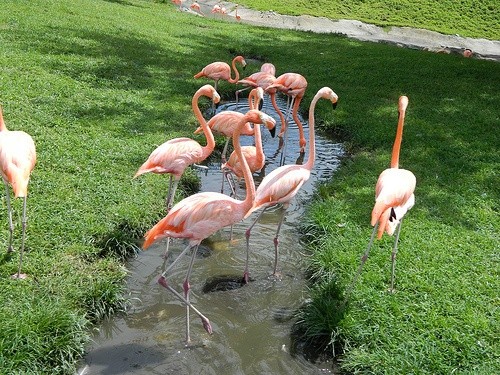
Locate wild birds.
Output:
[464,50,471,57]
[340,95,417,315]
[171,0,241,22]
[131,56,339,349]
[0,100,37,280]
